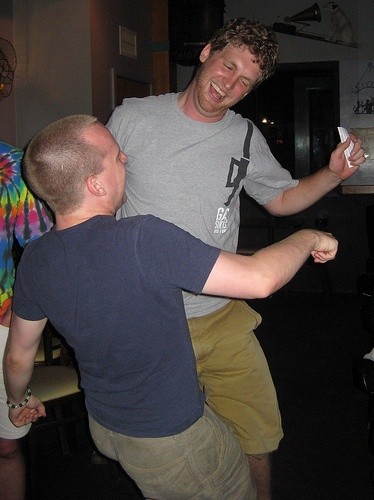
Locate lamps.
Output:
[266,3,324,36]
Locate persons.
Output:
[104,14,365,500]
[3,114,337,500]
[0,142,56,499]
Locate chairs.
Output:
[2,330,94,497]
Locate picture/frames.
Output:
[110,68,156,114]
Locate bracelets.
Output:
[6,387,32,409]
